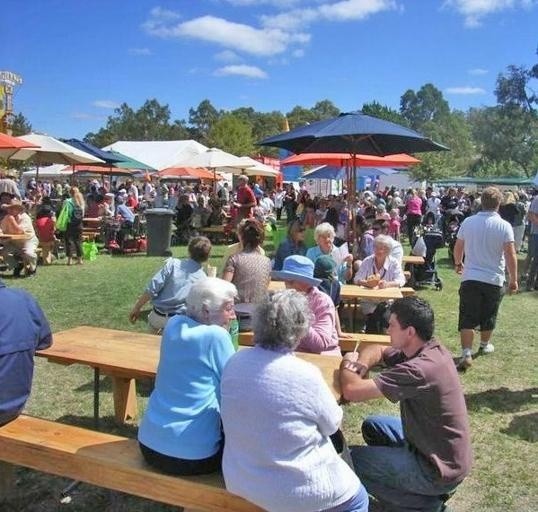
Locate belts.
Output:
[153,308,175,316]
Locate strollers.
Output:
[408,226,446,291]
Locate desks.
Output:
[198,202,238,245]
[0,198,141,278]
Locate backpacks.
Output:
[70,202,82,225]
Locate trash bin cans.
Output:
[143,208,176,256]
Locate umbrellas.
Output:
[278,152,422,192]
[253,110,452,221]
[0,131,282,196]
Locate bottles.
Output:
[228,320,240,352]
[337,261,347,283]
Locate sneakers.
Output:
[479,344,494,355]
[456,356,472,371]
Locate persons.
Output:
[0,173,537,292]
[269,255,343,359]
[340,295,472,512]
[129,236,212,335]
[222,218,272,333]
[137,276,239,475]
[219,287,369,512]
[0,276,53,428]
[454,186,519,370]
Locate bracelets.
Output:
[339,359,351,371]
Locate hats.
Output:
[1,199,25,210]
[315,256,336,273]
[270,254,323,285]
